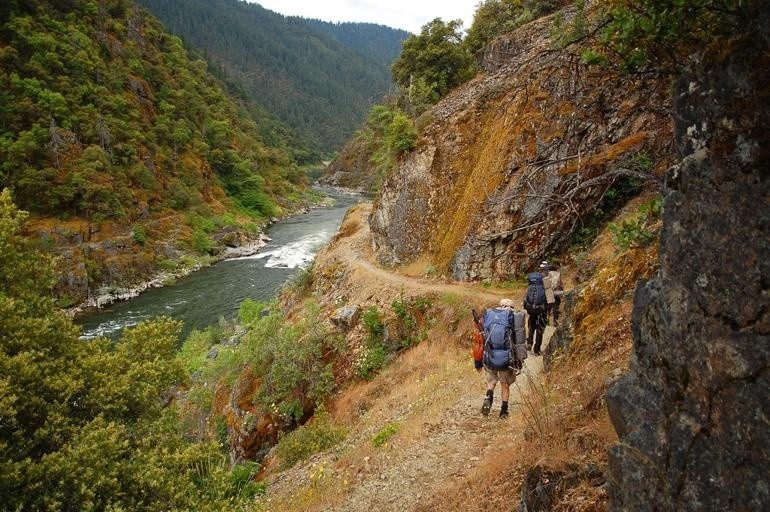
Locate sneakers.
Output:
[483,394,492,415]
[546,319,558,327]
[526,344,541,356]
[498,412,508,420]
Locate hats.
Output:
[539,261,547,268]
[499,298,516,307]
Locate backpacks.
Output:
[548,270,561,292]
[482,307,515,372]
[524,283,544,317]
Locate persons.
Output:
[525,259,564,356]
[547,264,563,327]
[474,298,522,419]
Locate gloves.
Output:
[513,367,522,375]
